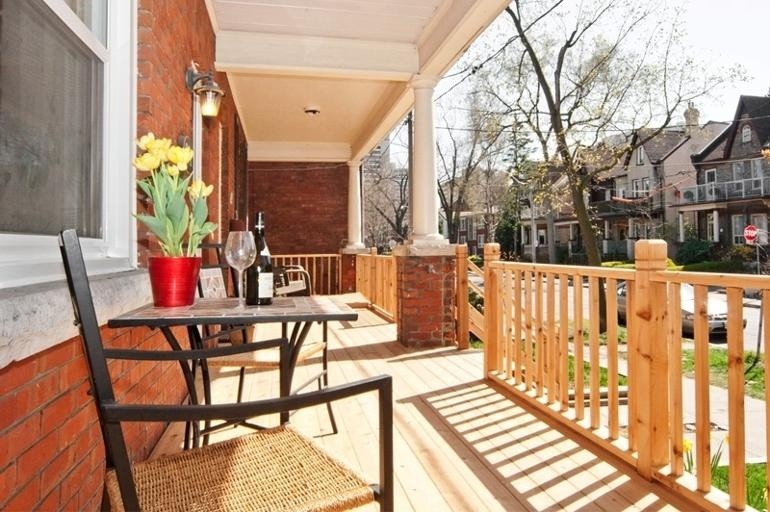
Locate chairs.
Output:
[181,240,339,433]
[57,225,395,512]
[230,219,312,296]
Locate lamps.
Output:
[185,65,226,118]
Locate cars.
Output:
[616,281,747,334]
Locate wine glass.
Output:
[225,230,257,310]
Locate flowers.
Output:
[129,132,220,258]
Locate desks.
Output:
[106,297,358,451]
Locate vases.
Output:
[144,256,204,308]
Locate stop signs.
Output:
[743,225,758,241]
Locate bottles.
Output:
[248,211,273,307]
